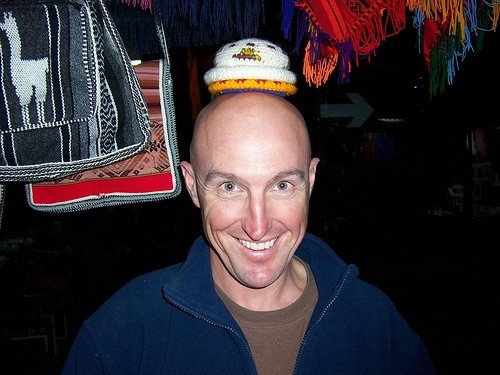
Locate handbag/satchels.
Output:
[0,0,152,180]
[23,60,181,211]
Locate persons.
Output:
[63,93,436,375]
